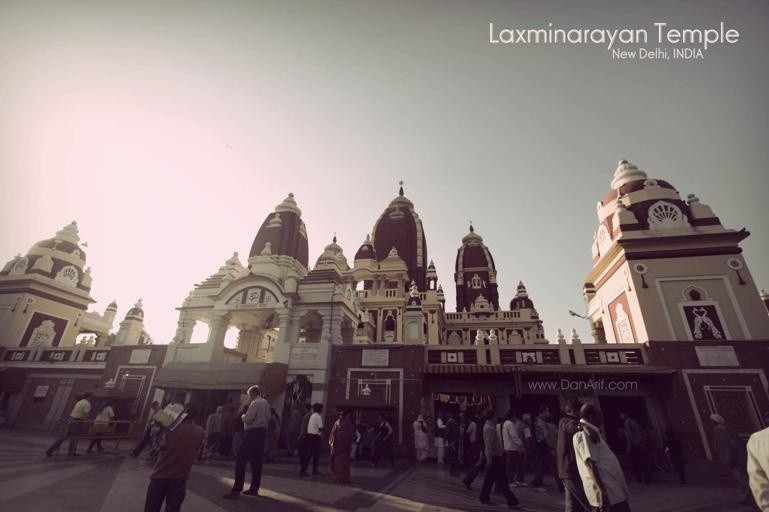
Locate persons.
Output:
[414,400,646,512]
[130,386,393,512]
[710,412,749,503]
[87,400,115,452]
[47,392,92,455]
[747,426,769,511]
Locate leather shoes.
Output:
[222,491,240,499]
[243,490,258,496]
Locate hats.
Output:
[710,414,725,424]
